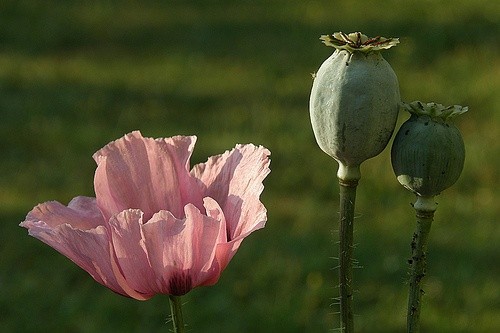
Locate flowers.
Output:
[18,129,273,333]
